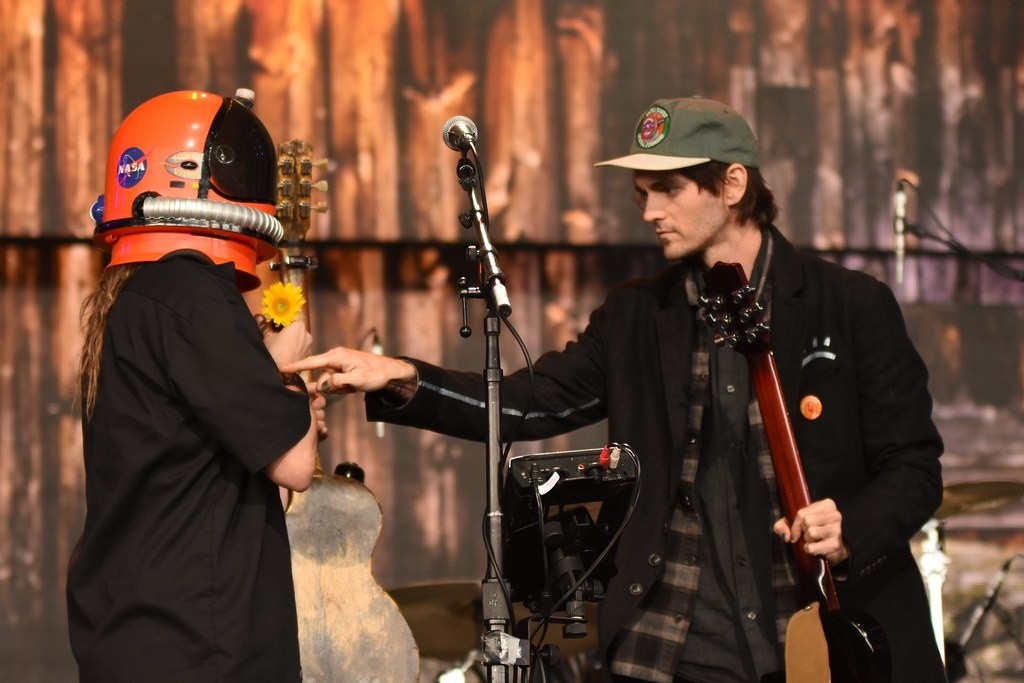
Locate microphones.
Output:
[443,116,479,151]
[892,183,909,284]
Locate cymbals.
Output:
[933,478,1023,519]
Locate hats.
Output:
[593,94,760,170]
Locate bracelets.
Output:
[281,373,308,394]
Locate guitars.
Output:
[698,259,881,683]
[268,135,423,683]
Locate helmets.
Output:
[92,87,285,295]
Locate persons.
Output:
[67,92,327,683]
[276,94,949,683]
